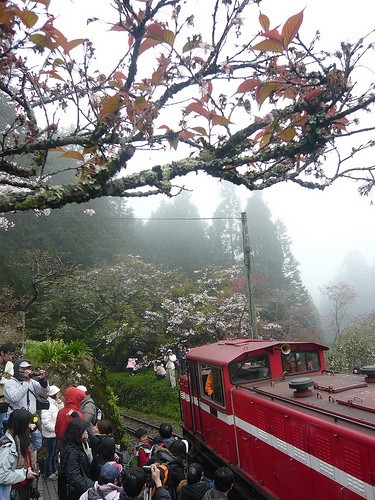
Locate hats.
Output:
[48,386,60,396]
[76,386,87,392]
[19,361,31,368]
[101,461,122,480]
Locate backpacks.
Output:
[82,401,101,427]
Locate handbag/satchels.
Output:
[82,441,93,464]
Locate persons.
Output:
[0,408,42,500]
[352,366,360,374]
[163,349,177,388]
[152,363,166,379]
[4,359,50,477]
[41,382,234,500]
[285,358,313,373]
[124,356,142,376]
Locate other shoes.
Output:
[55,470,58,474]
[49,474,58,480]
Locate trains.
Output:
[176,338,375,500]
